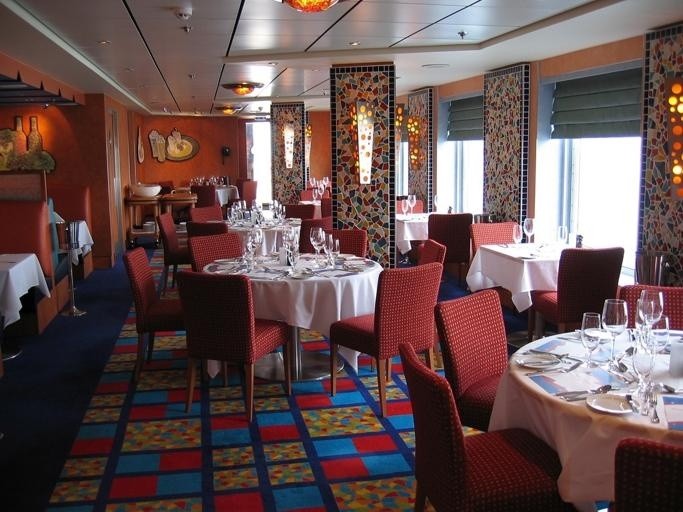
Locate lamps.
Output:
[663,71,683,201]
[406,114,420,171]
[355,101,375,186]
[304,122,313,168]
[55,220,94,317]
[213,106,241,115]
[284,121,294,171]
[395,102,405,177]
[273,0,340,13]
[221,83,260,97]
[223,147,230,157]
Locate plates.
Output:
[165,135,200,162]
[584,393,639,415]
[515,355,560,368]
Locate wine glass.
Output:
[580,289,682,394]
[511,217,576,250]
[401,195,442,219]
[188,175,376,277]
[56,221,88,318]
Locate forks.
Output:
[647,392,662,424]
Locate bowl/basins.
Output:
[132,184,161,197]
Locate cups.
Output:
[150,139,165,162]
[143,221,158,232]
[180,222,186,231]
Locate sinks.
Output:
[132,182,163,199]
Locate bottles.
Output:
[14,117,27,153]
[27,116,43,152]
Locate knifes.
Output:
[531,350,579,362]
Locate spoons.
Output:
[557,384,611,402]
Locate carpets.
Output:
[47,248,610,512]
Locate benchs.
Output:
[48,186,94,279]
[0,200,58,334]
